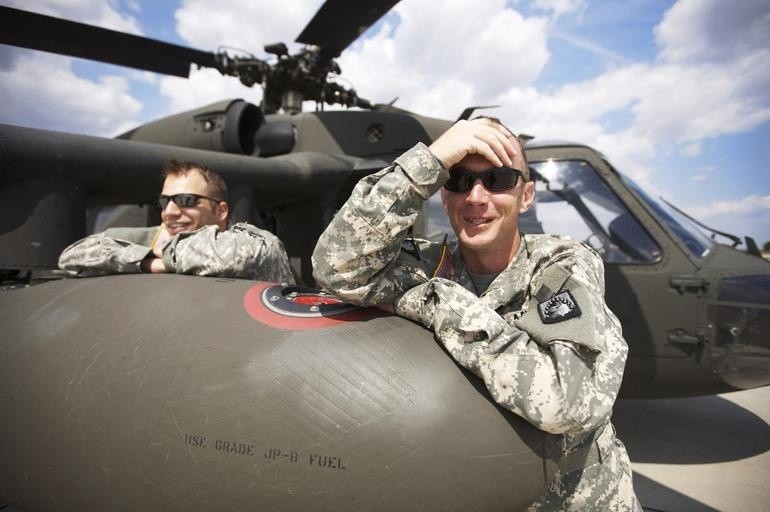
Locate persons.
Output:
[55,156,300,285]
[308,117,645,512]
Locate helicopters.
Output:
[0,0,769,399]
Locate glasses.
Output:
[155,193,222,208]
[443,166,527,193]
